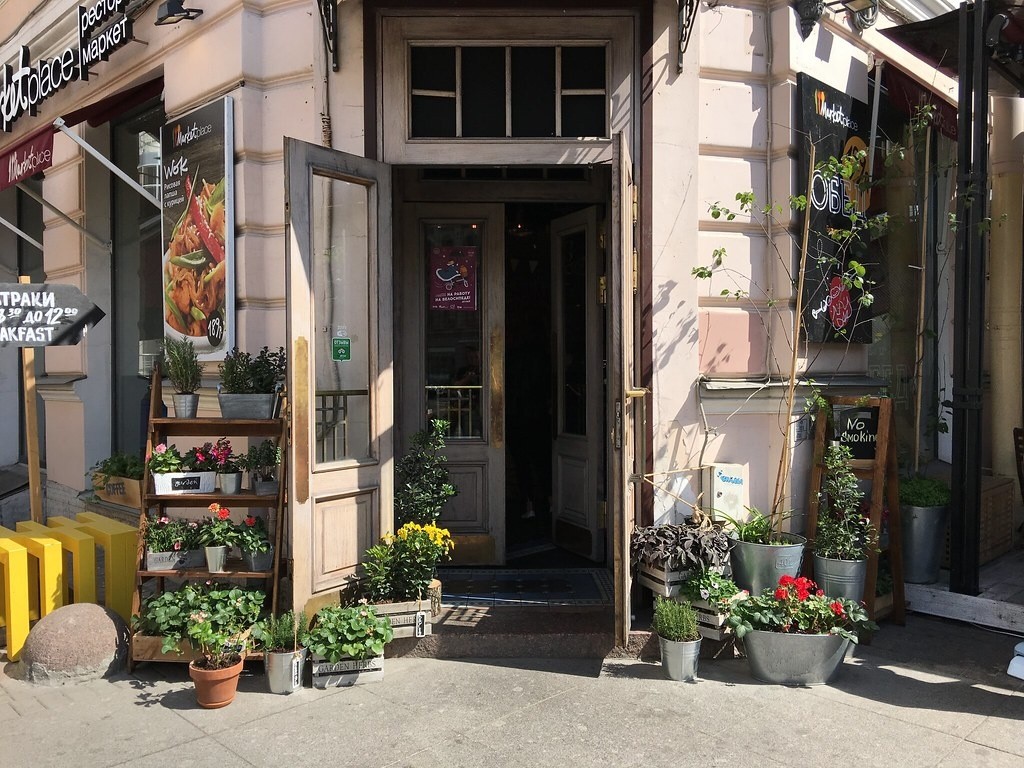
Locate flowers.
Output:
[686,569,741,600]
[721,574,868,634]
[145,443,211,472]
[206,501,231,547]
[243,515,266,545]
[185,580,253,659]
[327,599,375,647]
[380,521,454,599]
[209,436,234,472]
[153,513,211,551]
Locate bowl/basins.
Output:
[164,249,227,354]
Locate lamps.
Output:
[153,0,204,26]
[798,0,827,40]
[826,0,880,31]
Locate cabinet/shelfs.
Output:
[124,370,289,674]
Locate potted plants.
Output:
[243,439,282,496]
[813,442,882,606]
[212,436,243,494]
[143,515,206,571]
[260,604,311,695]
[217,346,286,419]
[727,575,880,686]
[394,418,461,617]
[74,453,145,509]
[302,600,395,688]
[188,603,246,708]
[236,514,275,571]
[146,442,217,495]
[653,595,703,681]
[629,515,733,599]
[345,523,454,638]
[861,90,1009,584]
[192,502,239,573]
[156,335,209,418]
[652,567,749,641]
[690,133,893,596]
